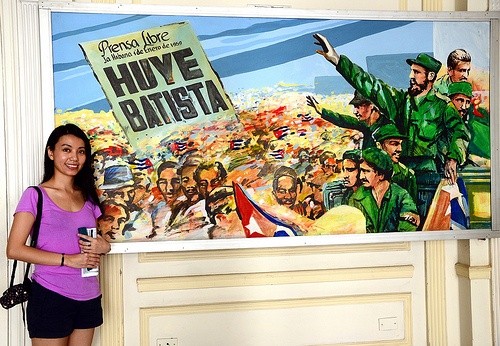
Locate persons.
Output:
[6,123,111,346]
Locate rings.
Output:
[89,242,91,246]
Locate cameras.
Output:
[0,284,30,309]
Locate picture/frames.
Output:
[37,0,500,253]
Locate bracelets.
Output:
[61,253,64,266]
